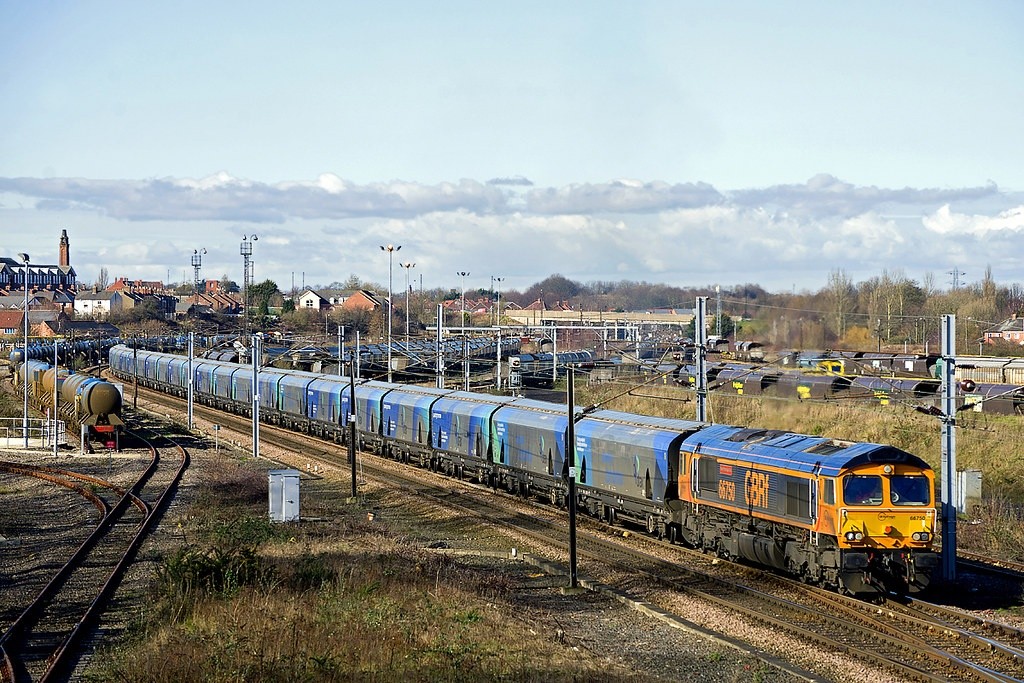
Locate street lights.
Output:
[239,233,258,339]
[379,243,401,383]
[454,272,471,333]
[398,262,418,350]
[493,276,505,325]
[15,253,31,448]
[191,248,208,324]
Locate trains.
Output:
[7,333,1024,601]
